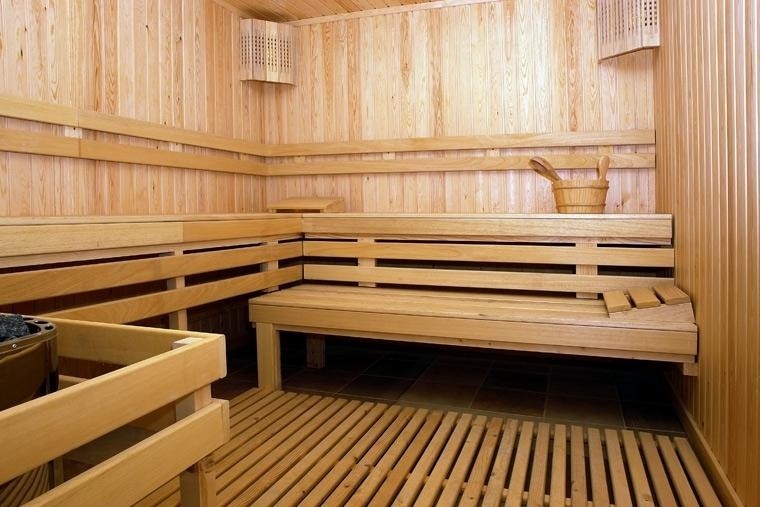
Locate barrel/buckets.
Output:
[552,158,609,213]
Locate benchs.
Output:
[248,213,698,391]
